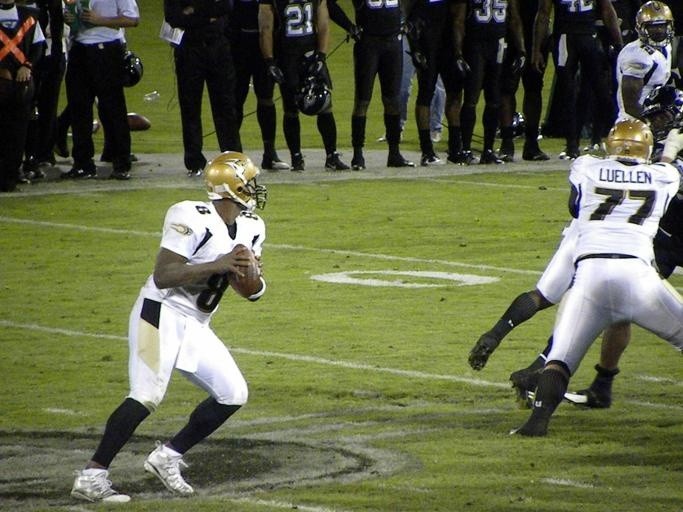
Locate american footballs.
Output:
[225,243,260,298]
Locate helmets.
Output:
[496,111,526,139]
[607,117,654,165]
[582,24,615,65]
[634,0,675,49]
[205,152,267,210]
[121,49,143,87]
[294,78,332,115]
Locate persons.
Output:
[469,1,682,437]
[161,1,683,173]
[1,0,153,194]
[69,149,268,507]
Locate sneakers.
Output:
[468,329,502,370]
[377,132,402,143]
[561,387,612,408]
[388,156,416,167]
[351,157,366,170]
[523,145,550,160]
[101,153,137,162]
[446,143,515,166]
[430,129,441,143]
[558,150,580,161]
[187,169,202,177]
[510,369,549,437]
[290,153,305,171]
[261,153,289,169]
[111,168,131,179]
[71,469,131,502]
[420,152,446,166]
[144,440,194,494]
[324,151,350,171]
[10,116,98,193]
[583,142,607,153]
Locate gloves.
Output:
[267,61,284,83]
[410,49,428,70]
[510,52,527,75]
[307,59,325,76]
[451,53,472,77]
